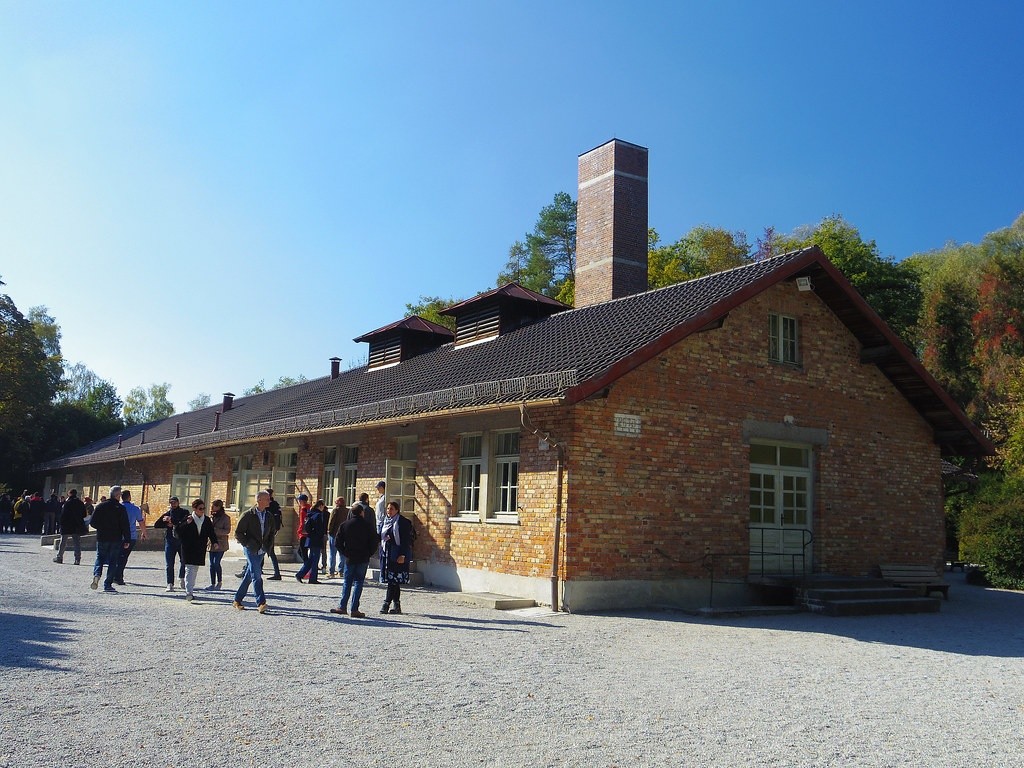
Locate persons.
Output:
[0,489,106,564]
[295,481,386,617]
[154,497,231,600]
[380,502,411,614]
[233,490,282,612]
[90,487,147,591]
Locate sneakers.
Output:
[232,601,244,609]
[259,602,267,613]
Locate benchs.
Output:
[878,563,950,601]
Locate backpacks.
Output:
[272,510,284,530]
[399,515,417,547]
[304,511,318,535]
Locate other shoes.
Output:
[165,576,222,601]
[53,557,62,563]
[309,580,321,584]
[103,586,117,592]
[351,611,365,617]
[267,575,281,580]
[74,560,80,565]
[235,573,243,578]
[296,574,303,583]
[91,576,99,589]
[331,608,348,614]
[114,579,125,585]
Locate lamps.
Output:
[796,276,815,292]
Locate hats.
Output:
[169,497,178,502]
[375,481,385,489]
[297,495,308,501]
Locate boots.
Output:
[390,601,401,613]
[380,600,391,614]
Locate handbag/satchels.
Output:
[172,514,193,540]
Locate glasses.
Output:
[197,507,206,511]
